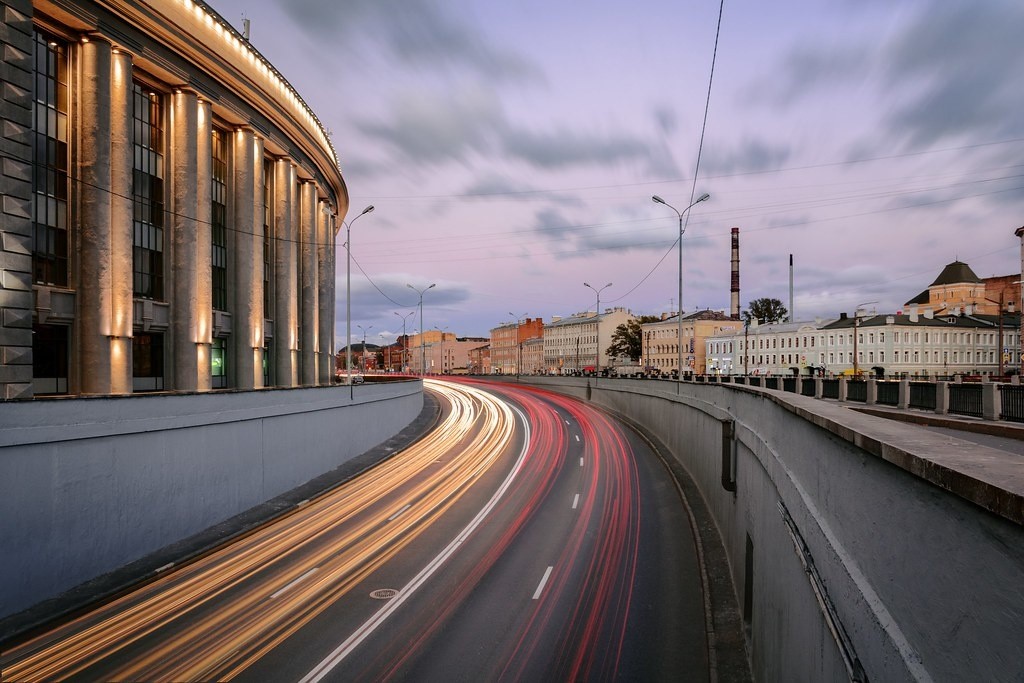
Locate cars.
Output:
[352,376,364,384]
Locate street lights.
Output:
[584,281,613,377]
[395,311,413,374]
[357,325,374,376]
[406,283,436,379]
[322,205,377,386]
[508,312,528,373]
[651,192,713,377]
